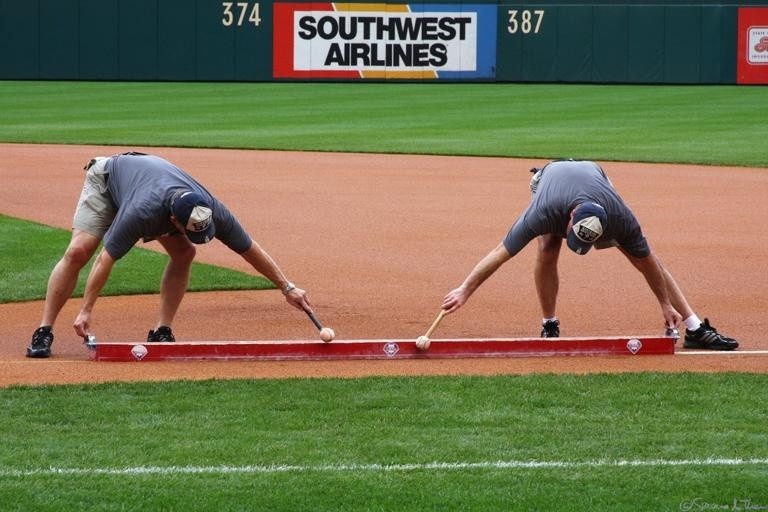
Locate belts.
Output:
[540,158,595,175]
[103,158,110,186]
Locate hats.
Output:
[565,201,609,256]
[168,188,216,246]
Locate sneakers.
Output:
[144,322,177,343]
[535,321,561,340]
[682,316,739,352]
[27,323,55,358]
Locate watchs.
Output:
[282,282,296,295]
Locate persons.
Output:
[440,157,740,352]
[23,149,314,359]
